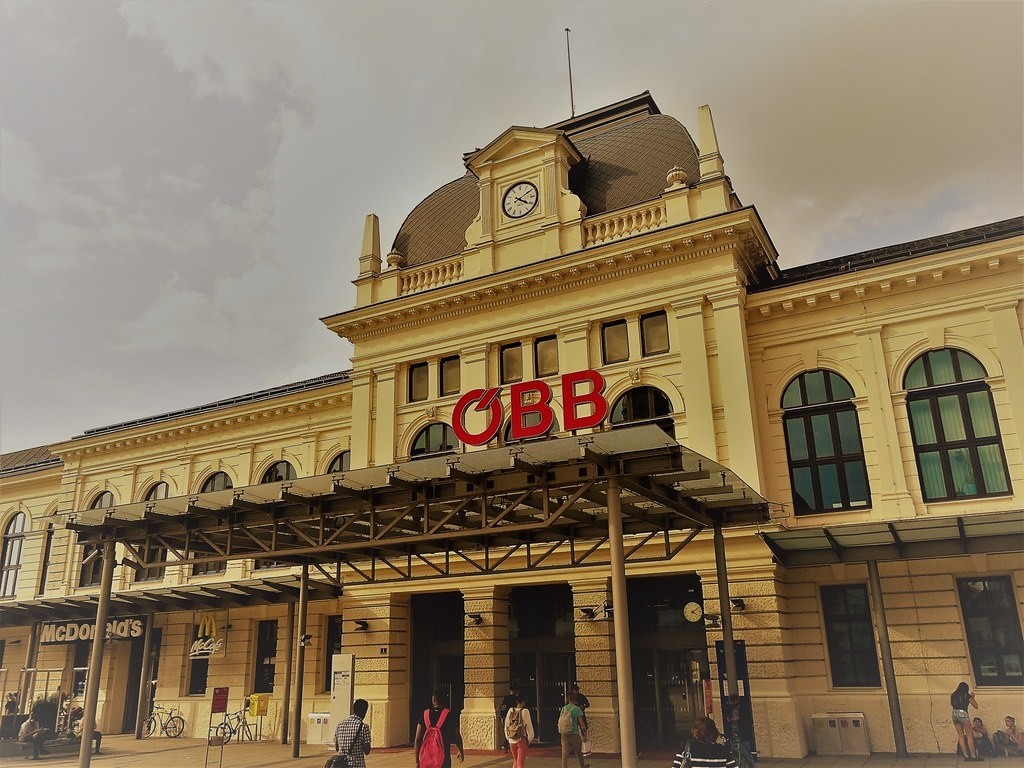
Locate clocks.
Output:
[683,601,702,622]
[501,182,538,218]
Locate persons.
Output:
[79,712,103,753]
[672,716,739,768]
[334,699,371,768]
[499,686,520,750]
[504,695,534,768]
[950,682,984,761]
[970,717,994,756]
[569,684,592,756]
[51,686,62,703]
[18,712,49,760]
[560,694,591,768]
[1001,716,1024,756]
[413,689,464,768]
[5,693,19,713]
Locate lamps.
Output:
[301,634,313,640]
[702,614,721,628]
[354,620,368,631]
[579,609,595,619]
[300,642,313,646]
[467,615,483,626]
[731,599,745,611]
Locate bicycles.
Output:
[140,704,186,739]
[215,703,252,744]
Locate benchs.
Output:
[15,737,93,760]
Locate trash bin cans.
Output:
[307,711,331,744]
[811,711,871,756]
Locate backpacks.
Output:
[558,706,576,734]
[419,708,449,768]
[507,706,527,740]
[993,731,1008,753]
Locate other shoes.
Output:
[40,750,49,754]
[33,756,42,760]
[964,755,984,762]
[95,751,101,754]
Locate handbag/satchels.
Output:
[324,755,348,768]
[581,740,591,756]
[680,741,692,768]
